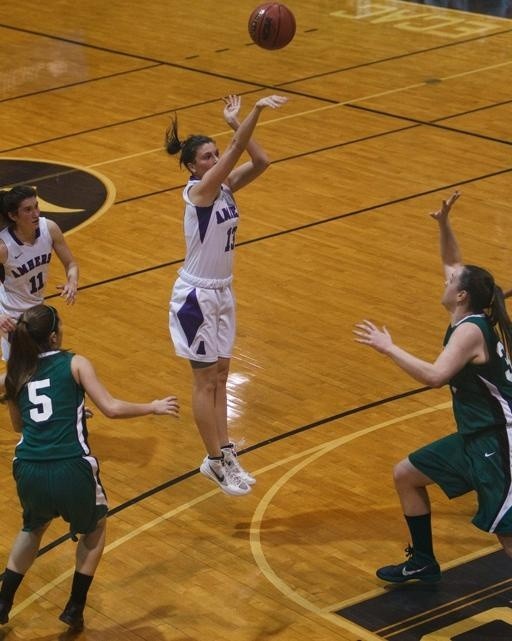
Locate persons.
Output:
[166,90,292,498]
[349,187,511,583]
[1,304,182,631]
[2,183,80,401]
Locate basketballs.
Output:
[249,0,297,49]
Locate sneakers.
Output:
[199,442,257,498]
[57,600,86,630]
[0,590,14,625]
[375,542,442,584]
